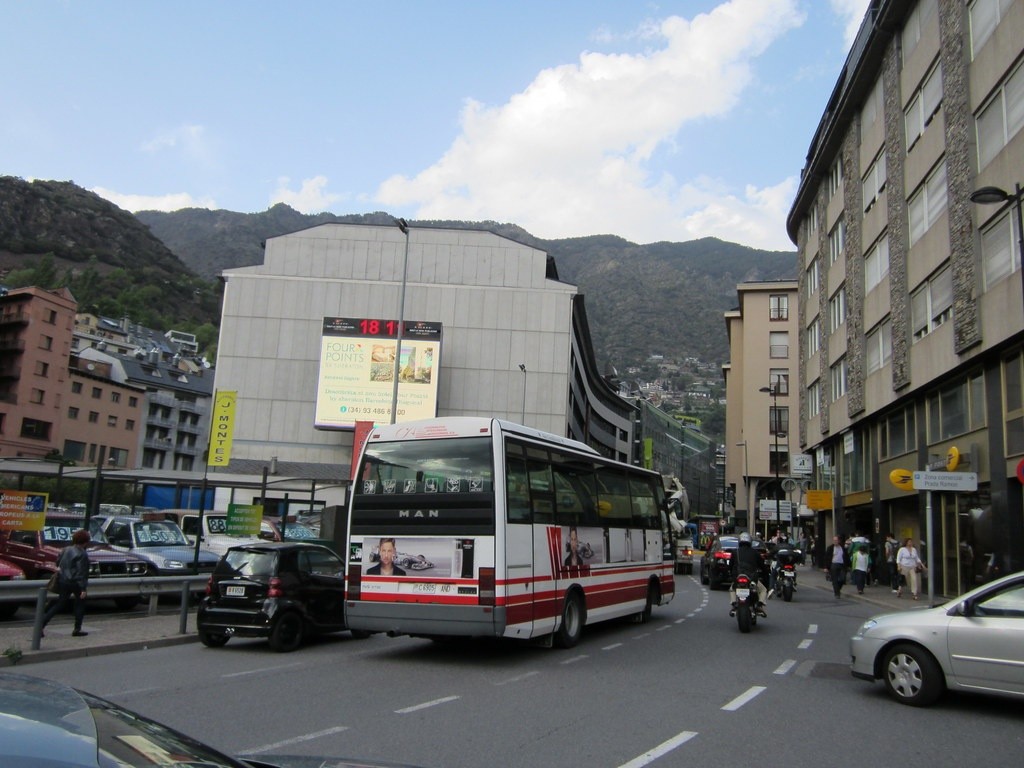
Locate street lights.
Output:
[715,451,725,535]
[759,386,780,531]
[736,440,750,535]
[518,364,527,425]
[391,217,410,424]
[971,181,1024,309]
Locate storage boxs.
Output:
[776,547,803,565]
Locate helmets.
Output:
[779,536,787,543]
[738,532,752,546]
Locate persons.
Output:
[365,538,406,576]
[39,530,91,637]
[565,527,583,566]
[774,532,925,600]
[954,539,973,562]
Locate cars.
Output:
[198,541,371,650]
[258,516,314,544]
[848,571,1024,707]
[0,560,26,614]
[0,670,419,768]
[699,534,770,590]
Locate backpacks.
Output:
[889,541,900,561]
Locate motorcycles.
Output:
[729,574,767,634]
[767,555,796,601]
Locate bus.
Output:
[342,416,677,653]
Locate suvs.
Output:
[154,509,260,568]
[88,515,223,604]
[0,514,148,612]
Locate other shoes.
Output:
[897,590,903,597]
[729,601,736,618]
[73,630,89,636]
[890,589,899,594]
[834,592,841,599]
[875,580,879,587]
[766,588,775,600]
[792,585,798,593]
[41,628,45,638]
[858,589,864,594]
[755,606,768,618]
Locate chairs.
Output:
[362,479,492,494]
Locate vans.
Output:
[673,522,697,573]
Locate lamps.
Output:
[270,455,278,474]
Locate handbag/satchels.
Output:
[914,565,924,573]
[46,572,61,593]
[737,567,758,580]
